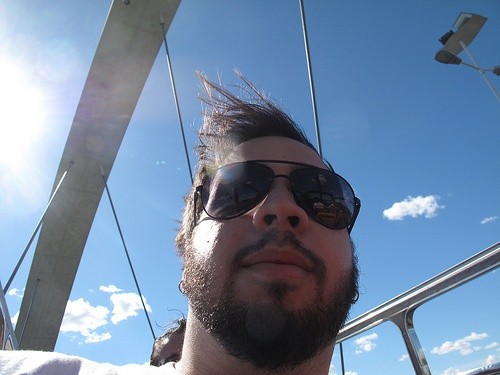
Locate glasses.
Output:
[191,156,360,235]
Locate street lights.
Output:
[434,12,500,103]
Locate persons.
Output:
[0,68,362,375]
